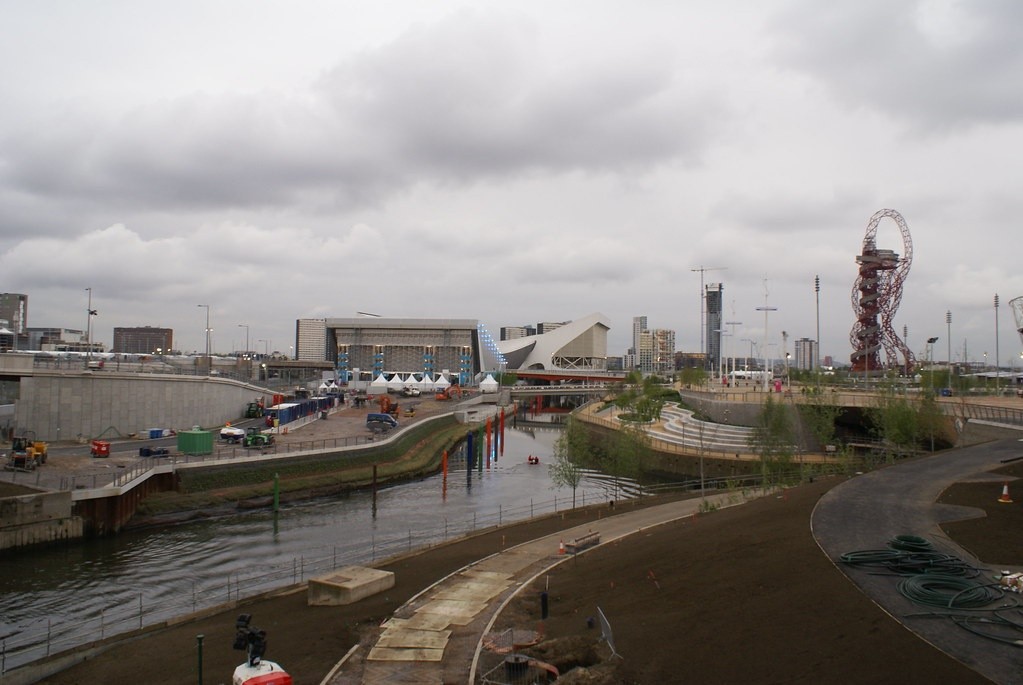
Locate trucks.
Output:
[399,387,421,396]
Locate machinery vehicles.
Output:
[435,383,461,401]
[4,431,48,473]
[245,396,266,418]
[242,427,275,449]
[404,404,416,417]
[380,395,400,418]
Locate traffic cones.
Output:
[558,539,566,556]
[998,480,1014,503]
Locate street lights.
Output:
[946,309,953,391]
[88,309,97,363]
[238,324,249,377]
[903,323,907,388]
[815,275,821,386]
[983,352,989,389]
[927,336,939,390]
[85,288,92,367]
[259,339,268,355]
[786,352,792,390]
[205,327,213,376]
[198,304,209,369]
[994,292,1000,397]
[681,420,688,451]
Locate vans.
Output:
[367,413,397,430]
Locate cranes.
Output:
[692,263,729,351]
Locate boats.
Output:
[529,457,539,465]
